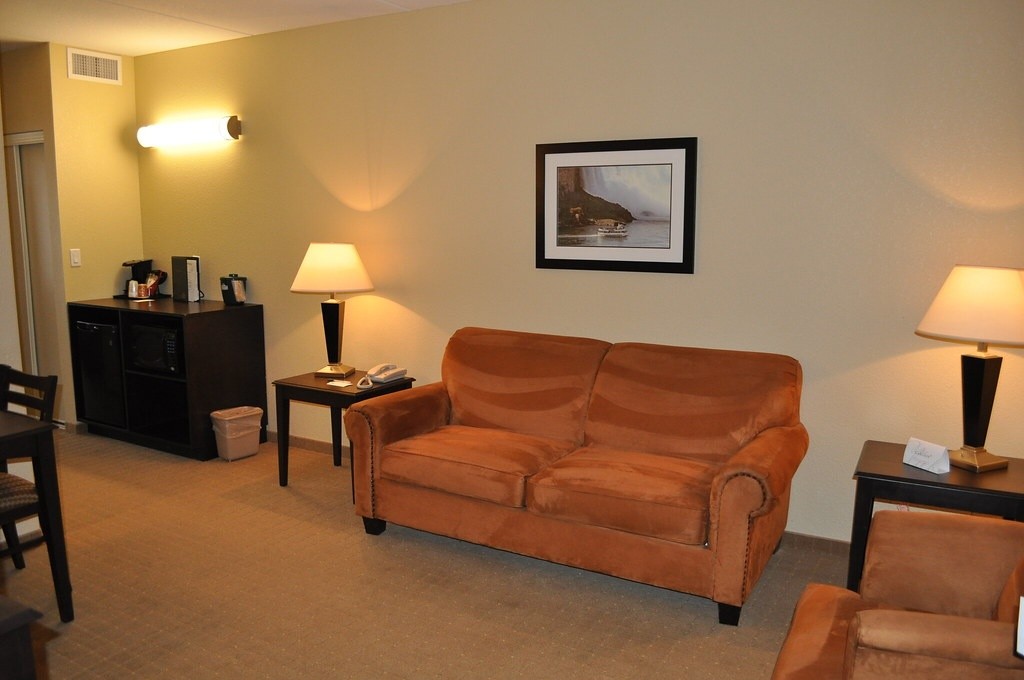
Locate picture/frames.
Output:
[535,137,698,274]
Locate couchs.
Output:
[770,510,1024,680]
[343,326,809,627]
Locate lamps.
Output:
[914,264,1024,474]
[136,115,241,149]
[290,243,375,379]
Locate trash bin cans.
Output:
[210,406,263,460]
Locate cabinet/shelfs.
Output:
[67,298,269,462]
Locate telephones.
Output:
[365,363,408,383]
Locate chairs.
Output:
[0,363,58,570]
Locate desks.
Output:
[846,439,1024,595]
[272,370,416,505]
[0,408,75,623]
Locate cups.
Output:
[138,284,150,296]
[129,281,138,297]
